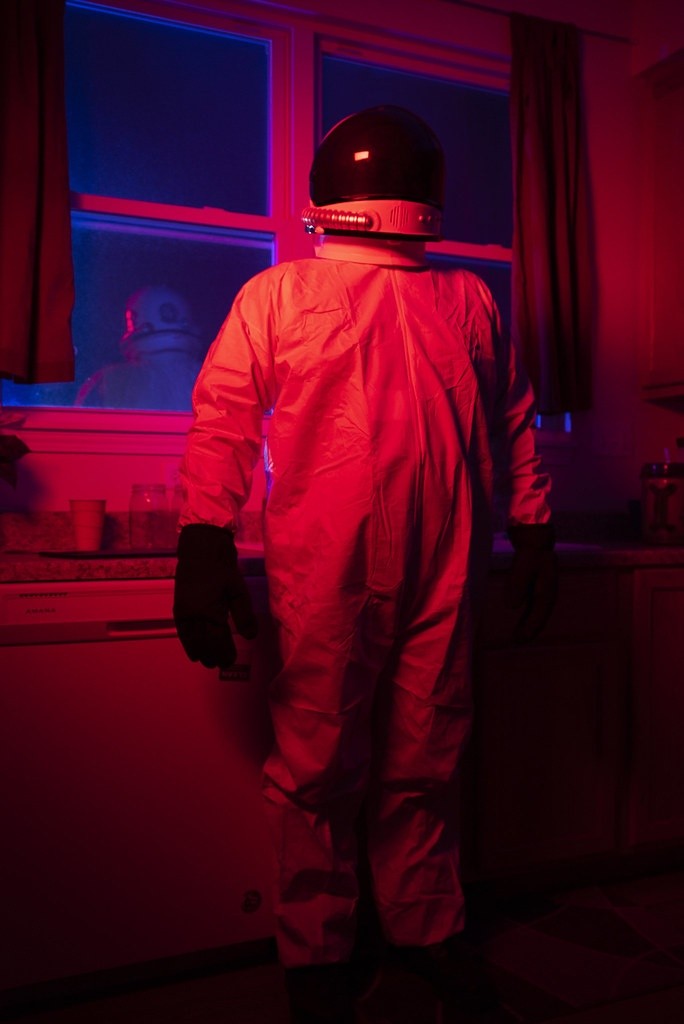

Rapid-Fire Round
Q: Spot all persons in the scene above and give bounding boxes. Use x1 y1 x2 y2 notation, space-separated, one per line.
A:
170 102 559 1024
74 282 208 413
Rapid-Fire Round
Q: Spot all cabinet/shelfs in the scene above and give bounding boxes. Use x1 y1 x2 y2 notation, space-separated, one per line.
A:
479 566 684 888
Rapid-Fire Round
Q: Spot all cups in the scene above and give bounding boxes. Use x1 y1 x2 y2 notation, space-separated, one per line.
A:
640 462 684 545
69 499 106 550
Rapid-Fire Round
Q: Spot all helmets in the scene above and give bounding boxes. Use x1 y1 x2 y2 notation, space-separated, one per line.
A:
300 105 448 241
115 281 207 363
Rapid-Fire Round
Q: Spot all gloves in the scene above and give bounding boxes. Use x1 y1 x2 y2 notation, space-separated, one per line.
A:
172 522 260 670
508 523 559 644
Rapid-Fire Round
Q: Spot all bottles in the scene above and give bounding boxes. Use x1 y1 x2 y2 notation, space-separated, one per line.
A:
128 484 170 551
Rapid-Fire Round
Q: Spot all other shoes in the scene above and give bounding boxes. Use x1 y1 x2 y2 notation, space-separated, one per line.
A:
401 940 507 1016
287 967 361 1024
